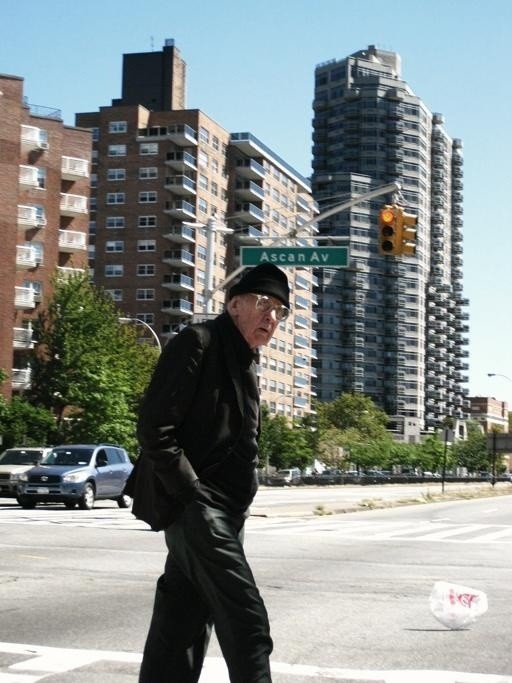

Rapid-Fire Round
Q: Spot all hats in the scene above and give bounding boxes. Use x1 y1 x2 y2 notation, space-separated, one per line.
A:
230 260 290 310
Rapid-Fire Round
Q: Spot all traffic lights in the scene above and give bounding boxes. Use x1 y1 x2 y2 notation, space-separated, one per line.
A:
402 211 418 256
380 209 398 253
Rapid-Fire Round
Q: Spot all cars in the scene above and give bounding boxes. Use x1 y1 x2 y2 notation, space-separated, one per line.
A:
266 466 443 487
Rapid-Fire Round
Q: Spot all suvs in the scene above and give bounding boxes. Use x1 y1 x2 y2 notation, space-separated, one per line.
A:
2 445 51 500
16 442 136 512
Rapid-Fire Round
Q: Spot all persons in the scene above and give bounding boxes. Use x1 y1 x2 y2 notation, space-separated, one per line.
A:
124 262 289 683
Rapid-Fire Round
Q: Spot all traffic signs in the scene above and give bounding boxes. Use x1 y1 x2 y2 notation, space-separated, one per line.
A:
240 244 351 266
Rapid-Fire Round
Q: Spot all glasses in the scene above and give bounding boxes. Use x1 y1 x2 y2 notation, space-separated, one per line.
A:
250 292 290 322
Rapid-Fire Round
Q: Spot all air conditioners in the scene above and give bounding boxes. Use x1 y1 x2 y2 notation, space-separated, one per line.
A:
37 141 50 151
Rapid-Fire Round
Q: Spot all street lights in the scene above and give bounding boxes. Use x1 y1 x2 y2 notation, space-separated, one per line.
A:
488 373 511 384
118 313 164 361
